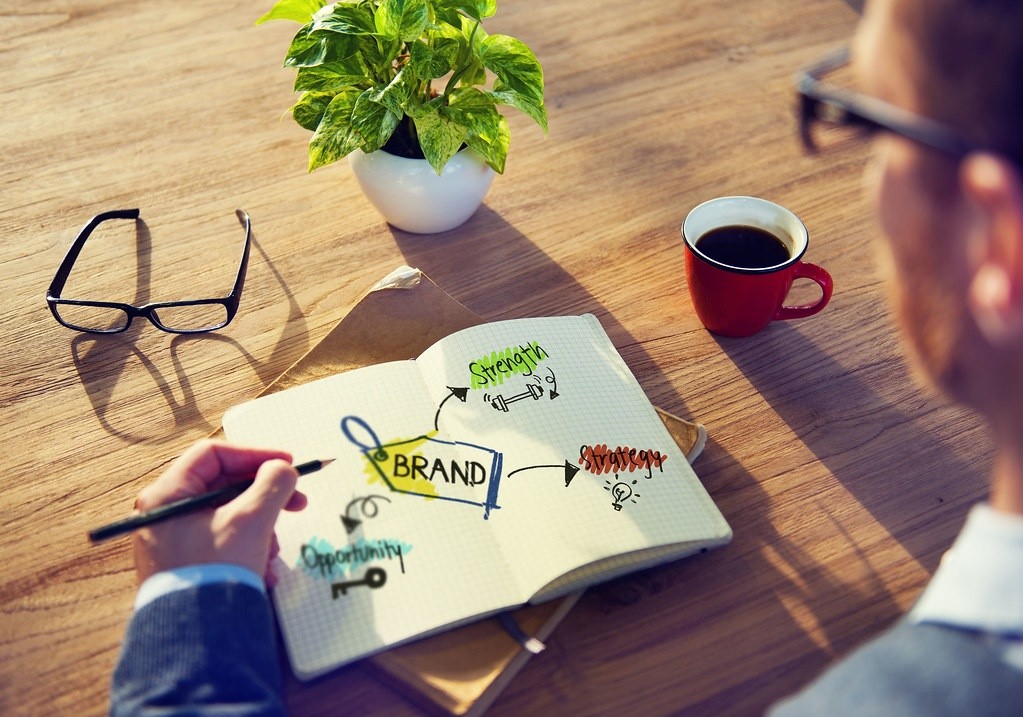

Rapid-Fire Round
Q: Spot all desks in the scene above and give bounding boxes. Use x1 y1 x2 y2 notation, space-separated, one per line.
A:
0 0 998 717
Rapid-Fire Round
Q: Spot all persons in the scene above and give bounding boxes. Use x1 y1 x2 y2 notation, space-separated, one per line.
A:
108 0 1023 717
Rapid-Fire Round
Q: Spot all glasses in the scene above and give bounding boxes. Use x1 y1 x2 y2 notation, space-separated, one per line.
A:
794 47 987 155
44 207 251 336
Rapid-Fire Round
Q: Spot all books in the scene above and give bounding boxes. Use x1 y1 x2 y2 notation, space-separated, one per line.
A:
133 265 734 717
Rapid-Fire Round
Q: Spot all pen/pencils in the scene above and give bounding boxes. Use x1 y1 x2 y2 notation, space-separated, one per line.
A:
87 458 338 543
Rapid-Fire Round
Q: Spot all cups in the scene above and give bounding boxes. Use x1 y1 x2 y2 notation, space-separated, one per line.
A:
681 196 833 338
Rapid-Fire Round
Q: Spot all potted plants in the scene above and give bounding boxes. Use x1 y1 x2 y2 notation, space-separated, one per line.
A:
254 0 548 233
